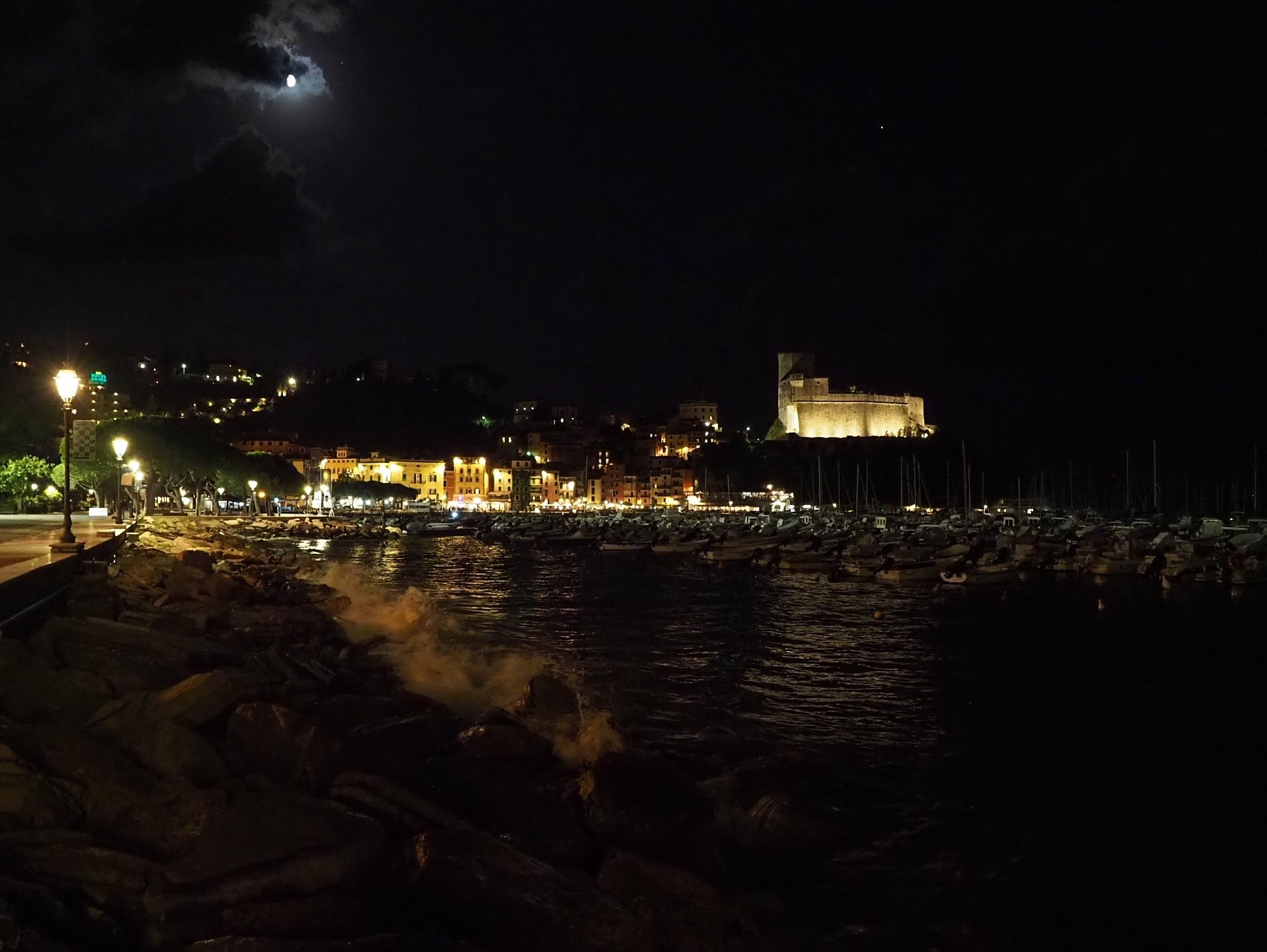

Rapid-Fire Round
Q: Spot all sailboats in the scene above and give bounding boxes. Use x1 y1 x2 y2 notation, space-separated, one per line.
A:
217 441 1266 597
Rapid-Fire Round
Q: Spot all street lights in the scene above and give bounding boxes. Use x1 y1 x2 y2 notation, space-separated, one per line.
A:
45 363 87 553
107 432 131 524
122 453 149 521
